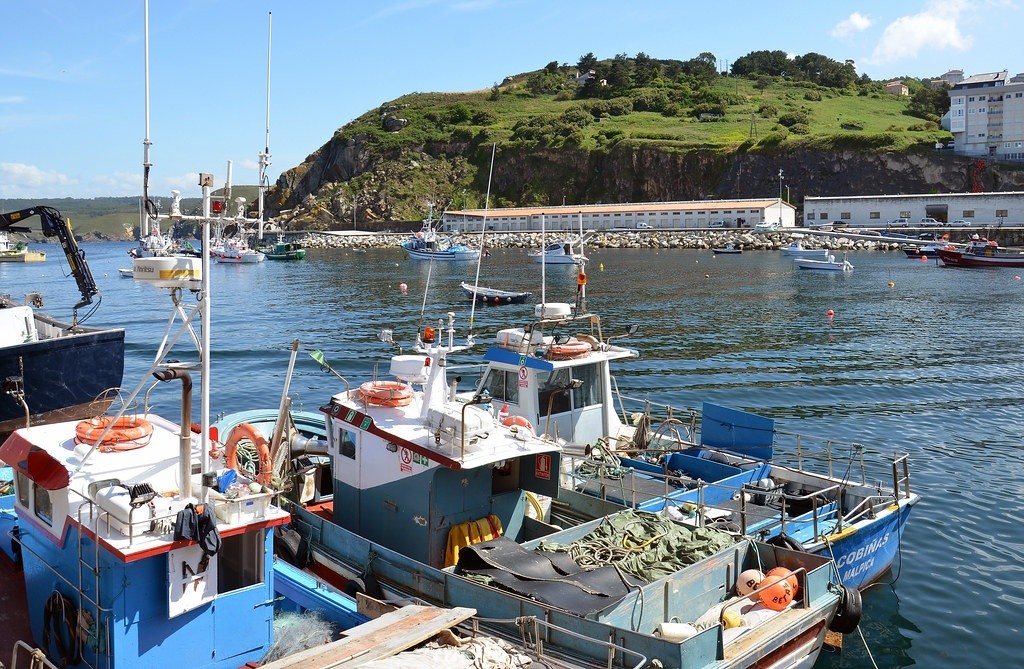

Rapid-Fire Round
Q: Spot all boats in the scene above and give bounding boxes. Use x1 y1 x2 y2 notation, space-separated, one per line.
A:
213 139 843 669
400 194 480 260
527 230 595 265
126 200 305 269
0 1 655 669
459 281 532 302
456 210 922 614
778 241 855 271
712 241 743 254
902 229 1024 268
0 231 46 262
353 247 367 253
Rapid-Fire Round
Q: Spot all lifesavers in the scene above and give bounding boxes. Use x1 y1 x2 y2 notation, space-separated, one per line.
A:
74 416 154 451
546 342 592 359
501 415 534 435
224 422 271 487
359 380 415 407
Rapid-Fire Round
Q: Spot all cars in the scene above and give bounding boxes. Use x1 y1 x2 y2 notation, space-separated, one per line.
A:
918 233 941 241
946 220 971 227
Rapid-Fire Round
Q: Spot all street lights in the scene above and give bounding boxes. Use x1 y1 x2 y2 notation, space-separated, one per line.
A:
778 169 784 227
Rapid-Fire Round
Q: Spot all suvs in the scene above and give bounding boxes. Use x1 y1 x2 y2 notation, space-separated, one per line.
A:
756 222 780 227
921 218 943 227
708 220 726 228
637 223 652 229
887 218 908 228
